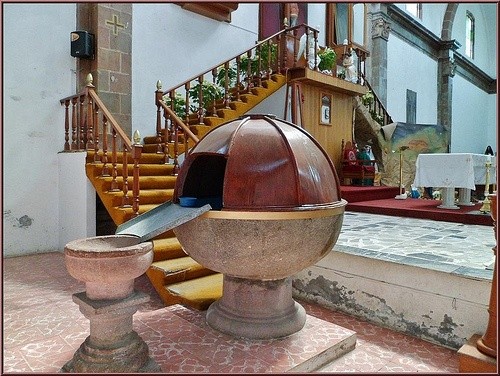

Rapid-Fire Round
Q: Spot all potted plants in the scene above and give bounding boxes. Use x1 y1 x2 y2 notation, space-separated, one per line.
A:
164 42 278 133
318 46 336 76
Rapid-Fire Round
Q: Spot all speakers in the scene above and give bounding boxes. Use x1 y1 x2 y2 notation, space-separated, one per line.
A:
71 31 93 59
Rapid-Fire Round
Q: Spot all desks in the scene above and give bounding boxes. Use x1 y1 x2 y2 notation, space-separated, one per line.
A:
413 153 496 209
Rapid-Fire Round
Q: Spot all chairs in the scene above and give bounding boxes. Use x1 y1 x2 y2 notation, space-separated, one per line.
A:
341 138 378 186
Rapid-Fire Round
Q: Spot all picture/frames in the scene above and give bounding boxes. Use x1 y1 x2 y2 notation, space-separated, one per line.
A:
319 91 333 126
